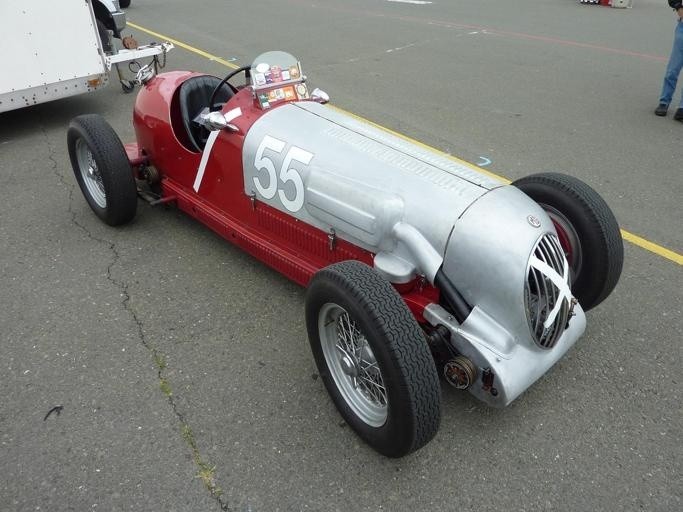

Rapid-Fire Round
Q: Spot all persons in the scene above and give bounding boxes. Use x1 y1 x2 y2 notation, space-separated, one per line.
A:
655 0 683 121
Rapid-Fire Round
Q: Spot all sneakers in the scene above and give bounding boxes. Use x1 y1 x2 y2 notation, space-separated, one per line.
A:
655 104 669 116
674 108 683 121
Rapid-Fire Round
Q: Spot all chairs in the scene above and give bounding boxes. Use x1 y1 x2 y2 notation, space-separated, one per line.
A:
179 74 233 154
